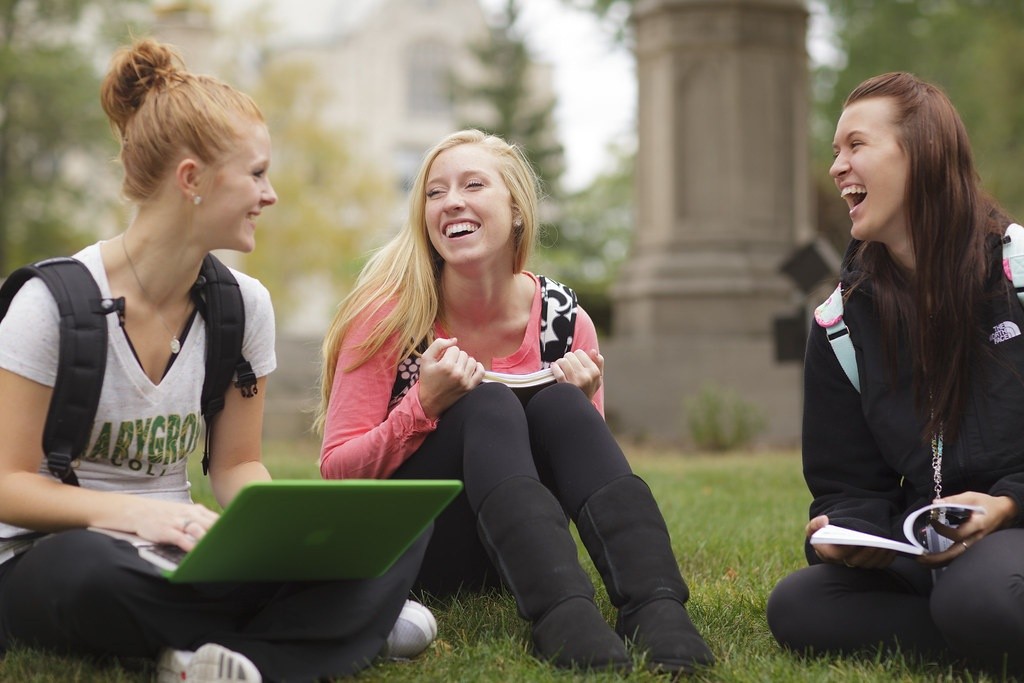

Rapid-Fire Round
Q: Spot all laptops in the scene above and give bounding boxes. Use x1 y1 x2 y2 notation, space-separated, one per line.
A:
84 481 466 586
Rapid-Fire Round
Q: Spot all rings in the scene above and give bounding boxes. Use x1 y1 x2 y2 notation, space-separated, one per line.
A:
179 517 194 531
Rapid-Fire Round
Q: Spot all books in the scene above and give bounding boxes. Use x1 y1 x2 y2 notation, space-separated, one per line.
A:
480 361 557 407
808 498 988 557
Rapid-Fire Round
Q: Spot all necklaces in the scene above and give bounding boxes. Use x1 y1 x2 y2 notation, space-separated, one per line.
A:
958 540 969 553
120 231 194 356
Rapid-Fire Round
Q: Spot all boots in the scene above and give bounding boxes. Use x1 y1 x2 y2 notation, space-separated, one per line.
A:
477 473 633 669
577 475 717 674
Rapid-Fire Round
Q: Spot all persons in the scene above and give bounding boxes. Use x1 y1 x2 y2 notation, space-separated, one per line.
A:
0 36 444 681
309 126 721 676
765 70 1024 676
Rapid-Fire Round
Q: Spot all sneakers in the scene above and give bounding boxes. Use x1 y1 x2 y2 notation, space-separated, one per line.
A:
377 598 440 659
156 642 263 683
